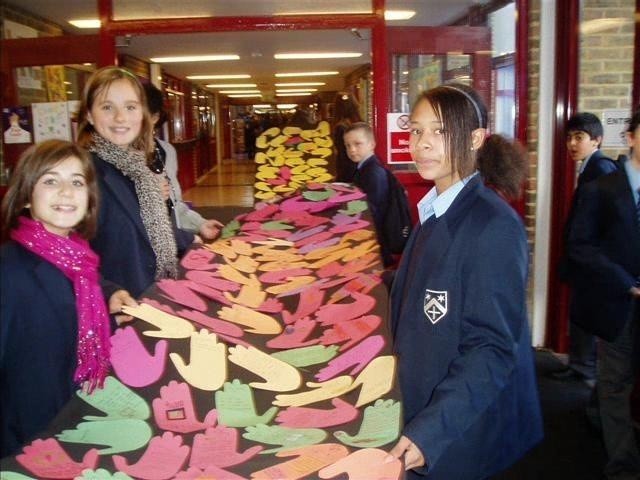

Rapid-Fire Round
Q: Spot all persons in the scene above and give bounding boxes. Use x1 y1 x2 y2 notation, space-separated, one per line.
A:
564 106 640 480
69 66 205 315
139 84 229 246
241 99 361 183
0 135 140 463
342 121 397 269
381 75 546 480
1 112 32 144
547 109 616 384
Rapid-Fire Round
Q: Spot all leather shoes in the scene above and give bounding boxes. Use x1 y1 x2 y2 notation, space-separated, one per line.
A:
550 367 581 379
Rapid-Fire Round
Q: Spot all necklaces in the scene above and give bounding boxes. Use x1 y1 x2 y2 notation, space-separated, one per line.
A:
143 144 180 212
